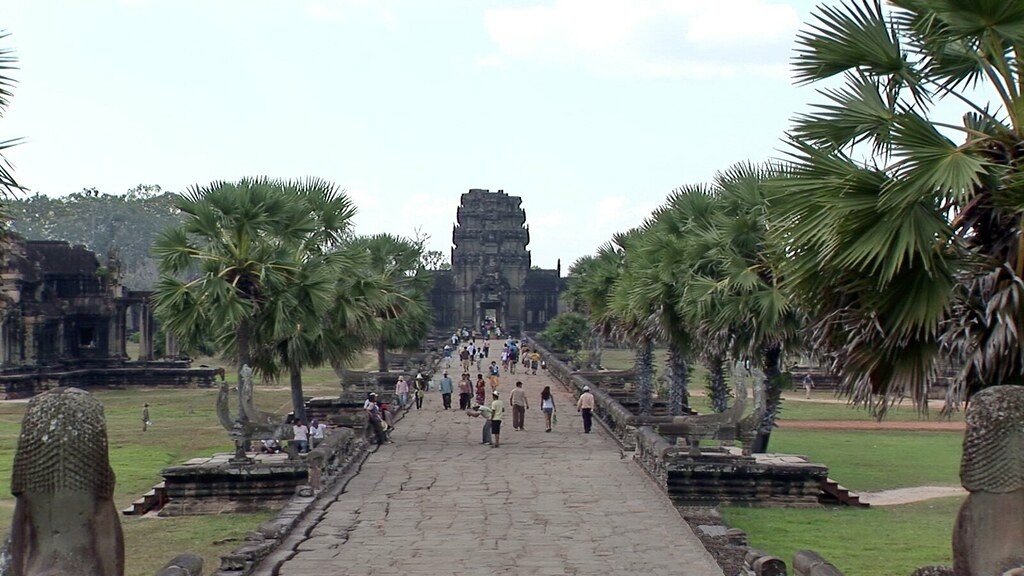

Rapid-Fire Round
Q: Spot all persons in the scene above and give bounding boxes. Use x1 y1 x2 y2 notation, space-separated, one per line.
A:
466 403 492 446
490 390 505 447
308 419 337 451
540 386 556 432
261 440 280 453
509 381 530 431
141 402 150 431
577 385 594 433
364 316 541 445
292 418 309 453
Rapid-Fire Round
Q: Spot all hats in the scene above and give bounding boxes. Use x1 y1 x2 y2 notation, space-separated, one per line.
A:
583 386 591 391
492 391 500 398
370 393 378 397
398 376 404 380
